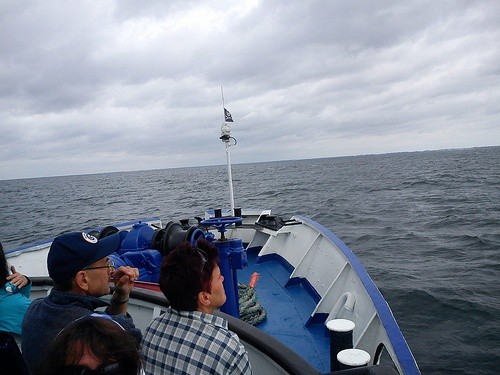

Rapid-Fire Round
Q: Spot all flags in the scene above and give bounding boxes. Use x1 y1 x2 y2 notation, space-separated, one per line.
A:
224 108 234 122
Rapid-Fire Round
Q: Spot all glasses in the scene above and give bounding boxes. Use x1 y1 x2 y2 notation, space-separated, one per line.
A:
194 246 209 273
83 260 115 272
60 361 128 375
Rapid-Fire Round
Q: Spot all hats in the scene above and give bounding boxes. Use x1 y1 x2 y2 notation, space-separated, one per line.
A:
47 230 129 278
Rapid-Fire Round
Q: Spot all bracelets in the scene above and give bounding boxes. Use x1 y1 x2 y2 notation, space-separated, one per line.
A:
111 297 130 305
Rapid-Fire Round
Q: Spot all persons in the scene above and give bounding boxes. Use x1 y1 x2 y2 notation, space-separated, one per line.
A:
142 242 253 375
0 243 33 374
21 230 143 374
38 314 141 374
